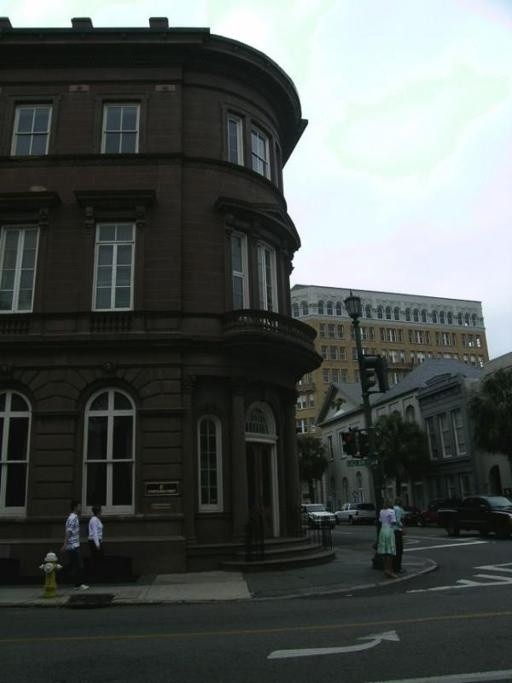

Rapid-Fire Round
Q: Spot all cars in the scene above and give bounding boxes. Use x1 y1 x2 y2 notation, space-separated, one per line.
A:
400 506 419 520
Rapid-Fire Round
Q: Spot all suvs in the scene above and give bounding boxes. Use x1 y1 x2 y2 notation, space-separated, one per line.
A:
415 499 454 527
334 502 378 525
301 504 337 530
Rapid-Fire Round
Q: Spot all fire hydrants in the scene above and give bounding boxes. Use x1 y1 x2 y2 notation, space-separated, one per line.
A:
39 552 63 598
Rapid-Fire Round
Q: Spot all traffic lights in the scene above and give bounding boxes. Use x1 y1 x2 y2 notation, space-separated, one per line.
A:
342 431 357 456
357 434 371 452
360 357 377 391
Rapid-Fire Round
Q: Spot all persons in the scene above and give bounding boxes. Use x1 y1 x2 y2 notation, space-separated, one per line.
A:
378 500 400 580
372 492 385 542
58 502 85 585
391 498 411 565
85 503 108 583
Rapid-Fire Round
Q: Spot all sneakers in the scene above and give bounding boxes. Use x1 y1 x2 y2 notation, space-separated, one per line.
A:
73 584 90 591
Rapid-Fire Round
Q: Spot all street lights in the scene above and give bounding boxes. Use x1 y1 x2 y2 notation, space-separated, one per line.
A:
343 289 385 570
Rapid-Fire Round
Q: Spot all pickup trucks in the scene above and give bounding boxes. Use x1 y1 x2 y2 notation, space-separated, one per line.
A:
437 495 512 538
